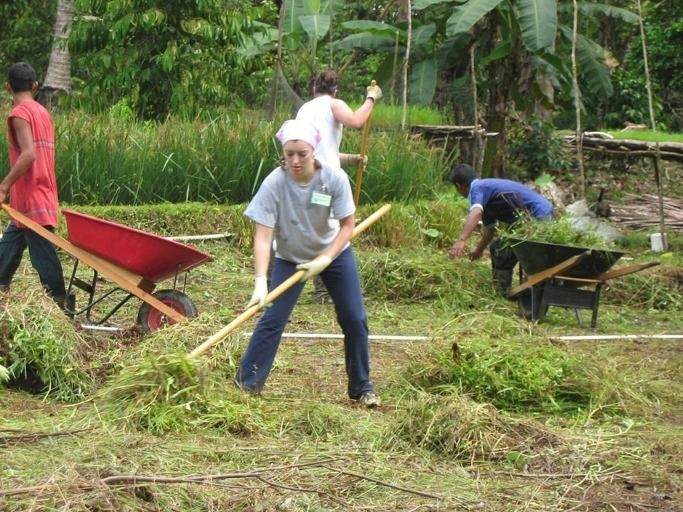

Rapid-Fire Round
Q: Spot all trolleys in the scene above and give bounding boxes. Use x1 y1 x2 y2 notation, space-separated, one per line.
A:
502 233 663 329
1 202 216 339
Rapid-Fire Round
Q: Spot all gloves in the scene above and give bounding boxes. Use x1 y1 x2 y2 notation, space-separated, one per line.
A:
247 272 268 310
348 153 368 167
295 253 332 281
367 84 382 102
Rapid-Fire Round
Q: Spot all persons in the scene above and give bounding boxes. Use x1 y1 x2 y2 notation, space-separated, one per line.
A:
233 119 381 408
448 164 554 296
0 62 69 316
297 72 383 302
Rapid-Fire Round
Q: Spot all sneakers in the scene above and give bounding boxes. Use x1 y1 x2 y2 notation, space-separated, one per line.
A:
362 388 380 407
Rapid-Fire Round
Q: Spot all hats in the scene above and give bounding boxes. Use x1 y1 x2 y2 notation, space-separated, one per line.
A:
277 119 322 150
